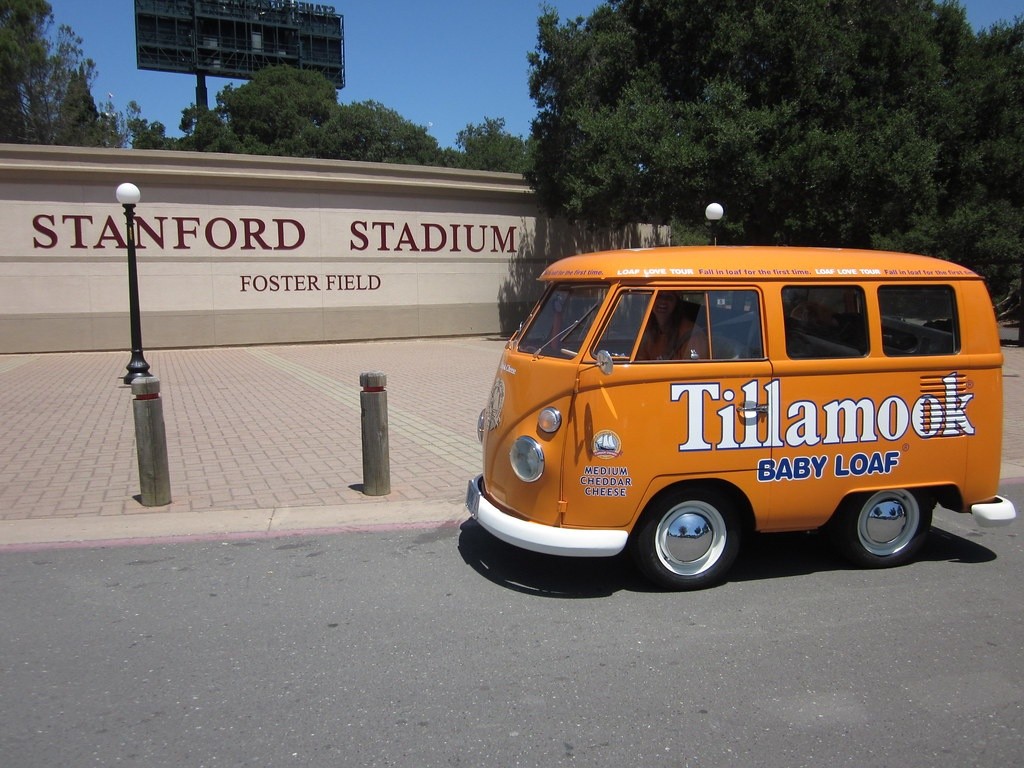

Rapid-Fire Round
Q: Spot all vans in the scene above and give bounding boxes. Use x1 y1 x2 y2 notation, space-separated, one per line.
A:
467 246 1005 593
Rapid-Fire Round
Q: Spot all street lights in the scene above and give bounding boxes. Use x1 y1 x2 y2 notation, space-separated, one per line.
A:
704 202 725 246
115 182 158 386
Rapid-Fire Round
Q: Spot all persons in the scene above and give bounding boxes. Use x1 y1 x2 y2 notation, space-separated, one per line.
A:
631 290 714 360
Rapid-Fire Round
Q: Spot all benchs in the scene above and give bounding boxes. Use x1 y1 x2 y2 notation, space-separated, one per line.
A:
786 329 860 359
848 312 954 356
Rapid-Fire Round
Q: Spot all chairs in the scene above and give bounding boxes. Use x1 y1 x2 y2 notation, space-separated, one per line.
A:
711 333 751 359
